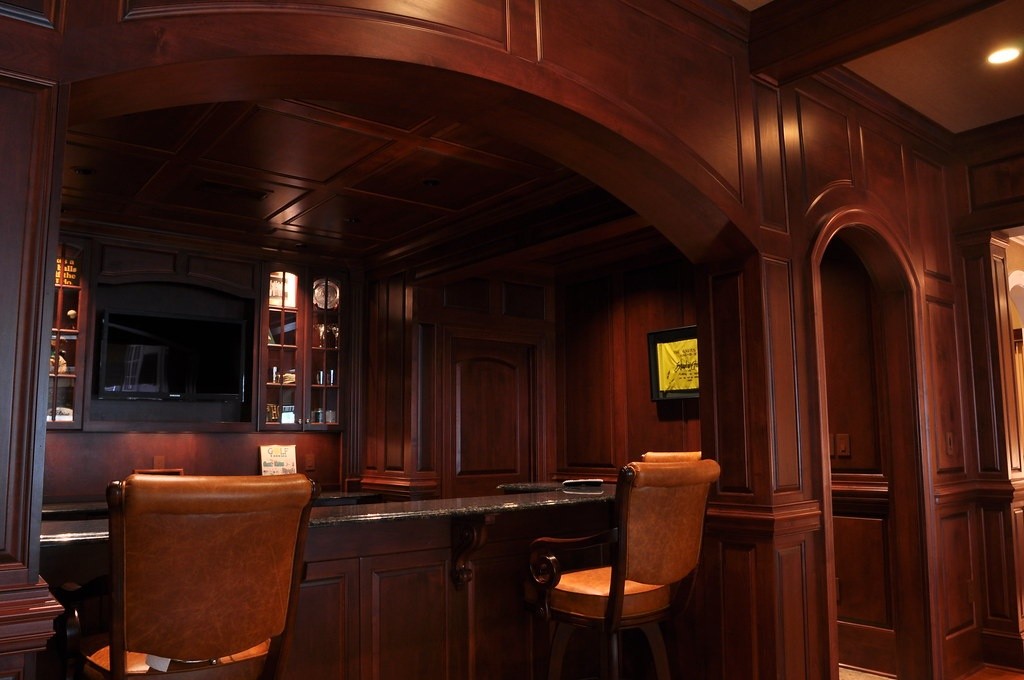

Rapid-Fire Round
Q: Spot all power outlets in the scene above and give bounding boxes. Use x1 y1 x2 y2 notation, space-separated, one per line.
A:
305 454 316 471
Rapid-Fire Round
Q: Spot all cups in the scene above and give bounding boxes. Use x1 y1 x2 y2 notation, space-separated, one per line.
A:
268 366 279 383
326 409 336 423
316 371 324 384
327 369 337 385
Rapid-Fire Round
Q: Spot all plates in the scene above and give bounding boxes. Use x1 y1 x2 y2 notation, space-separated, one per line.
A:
495 482 563 493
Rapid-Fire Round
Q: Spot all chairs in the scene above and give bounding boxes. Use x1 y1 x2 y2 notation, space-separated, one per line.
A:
526 460 722 680
54 472 323 680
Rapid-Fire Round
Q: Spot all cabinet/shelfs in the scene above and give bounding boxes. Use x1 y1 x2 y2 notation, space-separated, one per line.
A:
256 258 351 433
46 233 92 432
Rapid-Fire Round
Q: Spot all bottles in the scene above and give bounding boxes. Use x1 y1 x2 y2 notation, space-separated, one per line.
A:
315 407 324 423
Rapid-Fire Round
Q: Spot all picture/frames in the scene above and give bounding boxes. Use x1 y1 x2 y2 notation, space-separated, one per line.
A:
646 324 701 401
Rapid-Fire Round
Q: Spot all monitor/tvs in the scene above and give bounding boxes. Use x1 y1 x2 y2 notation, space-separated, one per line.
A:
97 309 246 402
